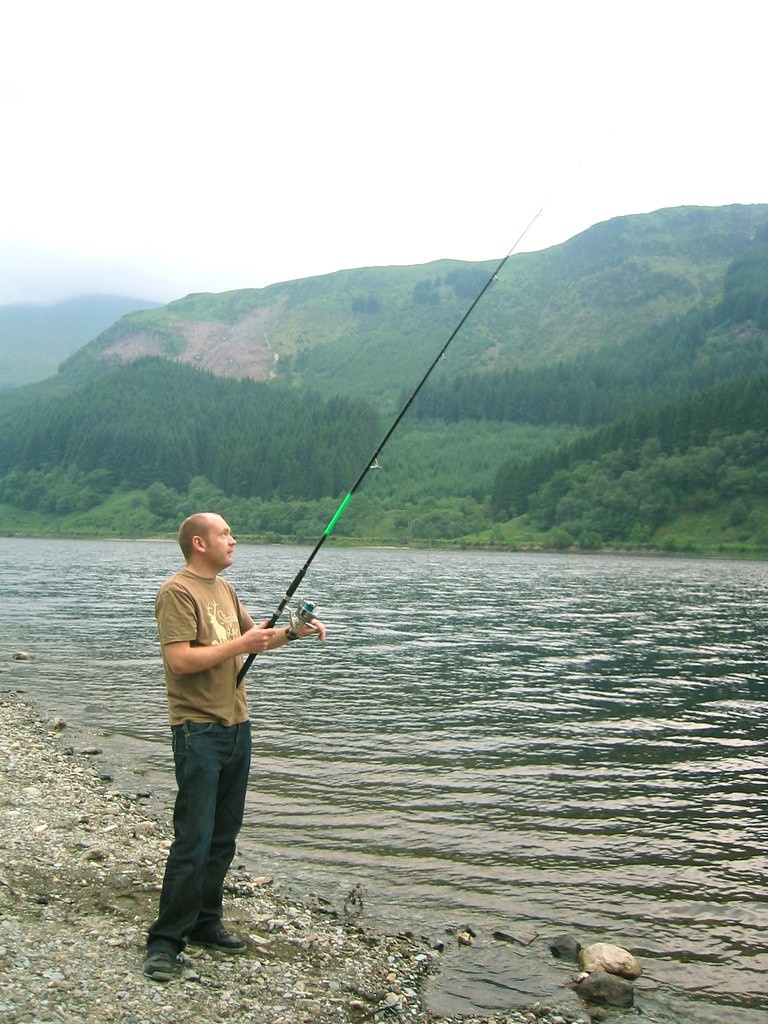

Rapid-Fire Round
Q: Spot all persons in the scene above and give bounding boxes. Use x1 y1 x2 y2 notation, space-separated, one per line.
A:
143 512 327 982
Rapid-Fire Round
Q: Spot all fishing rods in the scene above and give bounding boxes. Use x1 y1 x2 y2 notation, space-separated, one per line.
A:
237 204 548 690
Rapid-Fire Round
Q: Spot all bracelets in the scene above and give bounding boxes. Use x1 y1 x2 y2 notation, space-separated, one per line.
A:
285 624 300 641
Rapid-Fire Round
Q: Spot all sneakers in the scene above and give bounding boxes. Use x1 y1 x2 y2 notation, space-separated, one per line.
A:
143 952 174 981
184 924 246 954
